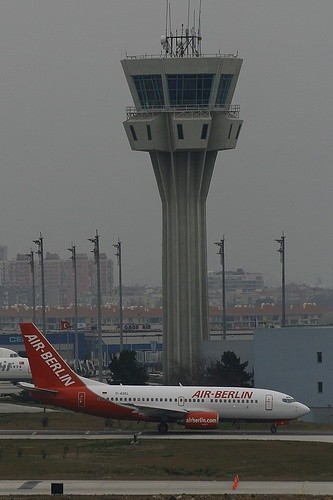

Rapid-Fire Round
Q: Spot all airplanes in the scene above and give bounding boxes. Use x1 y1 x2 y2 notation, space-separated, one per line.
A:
15 322 312 435
0 347 32 386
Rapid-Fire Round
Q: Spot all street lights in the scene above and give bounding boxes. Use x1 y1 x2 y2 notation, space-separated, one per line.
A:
213 234 226 339
31 232 46 337
25 250 36 324
274 230 285 328
66 240 79 374
111 235 123 353
87 229 103 383
